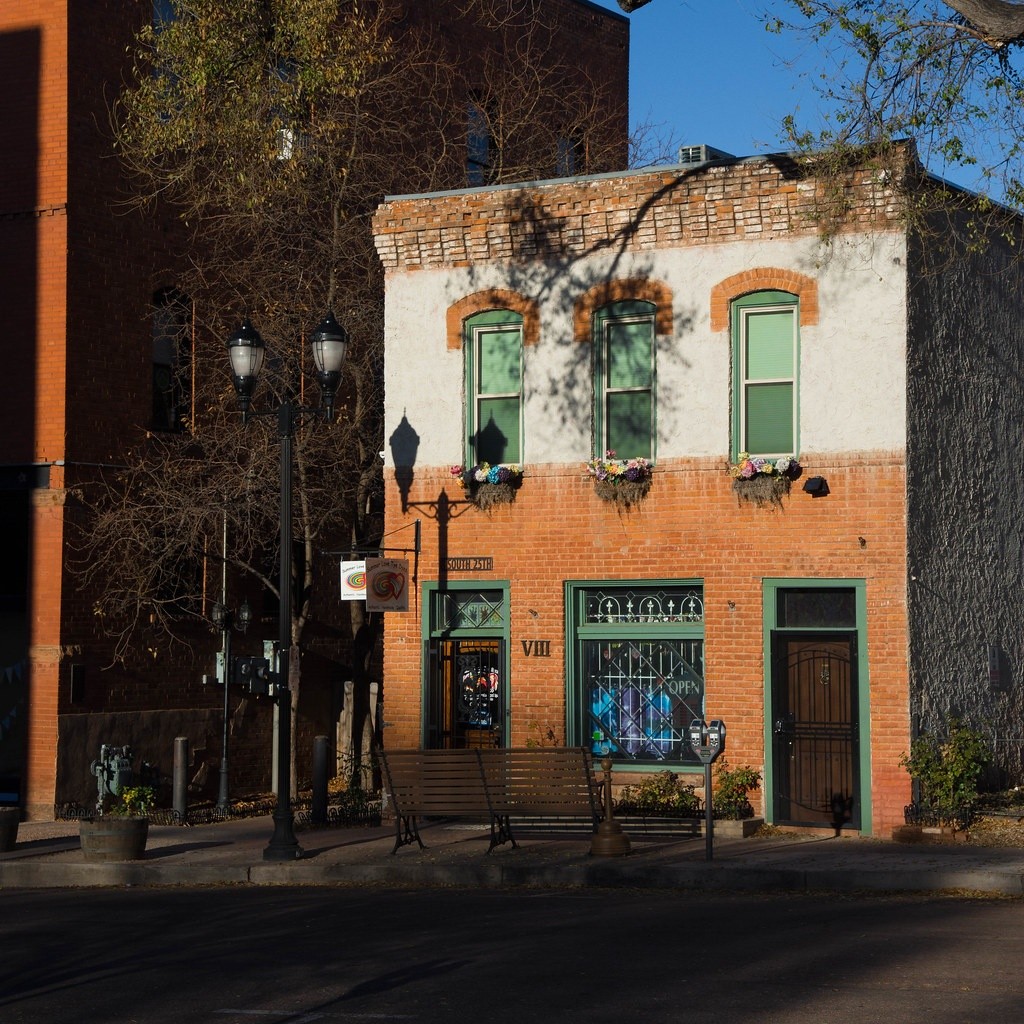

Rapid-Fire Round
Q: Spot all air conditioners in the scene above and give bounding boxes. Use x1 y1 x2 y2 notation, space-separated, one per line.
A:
678 145 737 165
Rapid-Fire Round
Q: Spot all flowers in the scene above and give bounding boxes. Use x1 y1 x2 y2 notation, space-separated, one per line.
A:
106 784 154 816
588 451 655 484
451 464 527 490
731 452 799 481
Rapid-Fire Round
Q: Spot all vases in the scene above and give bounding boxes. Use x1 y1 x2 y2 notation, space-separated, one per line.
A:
78 813 147 862
0 807 21 848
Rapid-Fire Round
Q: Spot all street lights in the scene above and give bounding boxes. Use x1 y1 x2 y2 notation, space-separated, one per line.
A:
224 304 351 866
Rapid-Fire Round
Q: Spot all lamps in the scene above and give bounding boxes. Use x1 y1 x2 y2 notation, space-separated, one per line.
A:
802 476 826 494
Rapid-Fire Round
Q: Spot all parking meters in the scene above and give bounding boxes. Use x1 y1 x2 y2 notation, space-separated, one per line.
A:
694 718 730 872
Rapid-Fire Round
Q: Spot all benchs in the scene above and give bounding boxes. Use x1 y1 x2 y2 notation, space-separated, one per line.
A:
374 744 611 855
